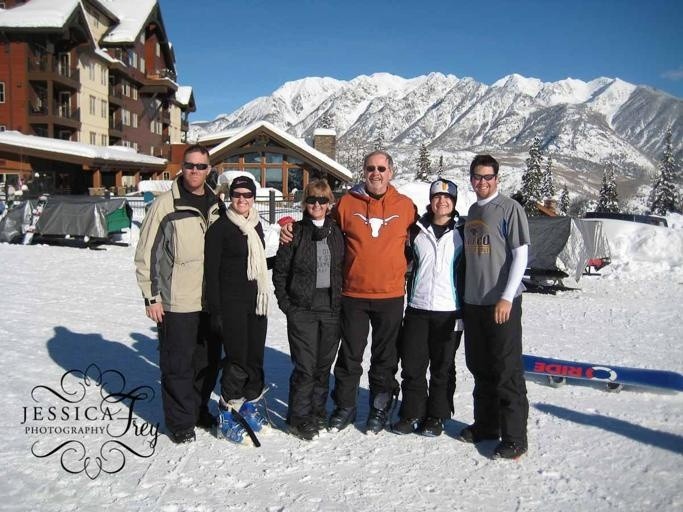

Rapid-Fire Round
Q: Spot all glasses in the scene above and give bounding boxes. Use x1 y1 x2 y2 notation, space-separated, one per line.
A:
305 196 329 204
472 173 496 181
184 163 207 170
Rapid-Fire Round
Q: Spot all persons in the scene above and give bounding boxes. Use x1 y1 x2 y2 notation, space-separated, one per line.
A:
135 145 228 443
458 155 531 461
278 148 419 435
276 177 345 439
205 175 281 445
391 178 466 438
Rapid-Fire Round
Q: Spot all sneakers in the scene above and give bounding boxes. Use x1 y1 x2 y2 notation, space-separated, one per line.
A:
328 405 358 432
197 411 218 428
288 419 320 440
217 395 252 446
420 416 446 437
390 416 426 435
311 414 328 430
239 399 273 434
366 408 389 435
174 426 197 444
494 435 528 459
460 423 500 443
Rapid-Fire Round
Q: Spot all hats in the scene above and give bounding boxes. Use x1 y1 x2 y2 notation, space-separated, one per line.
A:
429 179 457 200
229 176 256 197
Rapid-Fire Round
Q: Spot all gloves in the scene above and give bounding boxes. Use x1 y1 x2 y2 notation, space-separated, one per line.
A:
207 313 226 340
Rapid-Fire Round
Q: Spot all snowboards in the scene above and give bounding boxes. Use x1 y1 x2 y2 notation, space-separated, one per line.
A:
522 355 682 391
